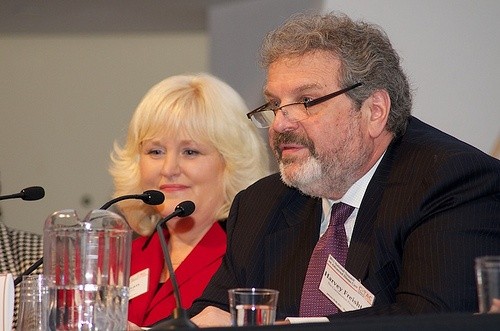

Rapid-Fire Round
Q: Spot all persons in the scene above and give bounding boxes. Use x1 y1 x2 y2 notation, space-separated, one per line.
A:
50 74 268 331
127 10 500 331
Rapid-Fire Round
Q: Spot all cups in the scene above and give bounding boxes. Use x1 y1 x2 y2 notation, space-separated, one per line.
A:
228 288 279 327
475 257 500 314
17 275 57 331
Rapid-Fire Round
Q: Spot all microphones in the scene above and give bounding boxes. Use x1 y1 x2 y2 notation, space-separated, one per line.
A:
147 201 200 331
14 190 165 284
0 186 45 201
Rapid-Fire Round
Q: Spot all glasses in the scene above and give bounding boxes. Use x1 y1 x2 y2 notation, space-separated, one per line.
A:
246 82 363 128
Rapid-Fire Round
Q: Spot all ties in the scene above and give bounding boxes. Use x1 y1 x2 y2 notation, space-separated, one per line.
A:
299 203 355 317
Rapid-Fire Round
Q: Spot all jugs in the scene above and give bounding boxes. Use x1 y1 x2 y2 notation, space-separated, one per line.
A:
41 209 132 330
